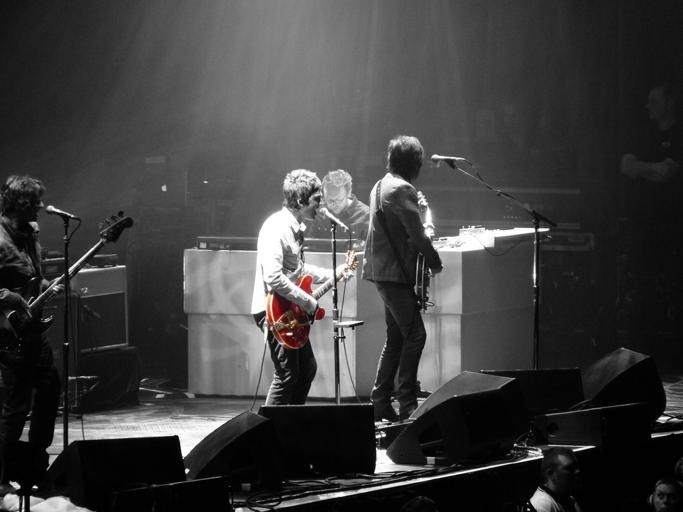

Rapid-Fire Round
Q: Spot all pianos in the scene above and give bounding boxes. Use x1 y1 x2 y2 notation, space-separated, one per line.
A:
195 236 362 252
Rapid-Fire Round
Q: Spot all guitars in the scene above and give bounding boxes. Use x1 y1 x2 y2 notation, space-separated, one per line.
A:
2 210 137 371
267 252 358 348
414 204 435 309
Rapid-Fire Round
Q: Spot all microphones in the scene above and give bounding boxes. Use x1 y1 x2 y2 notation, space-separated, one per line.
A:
429 153 462 162
46 205 79 222
320 208 351 231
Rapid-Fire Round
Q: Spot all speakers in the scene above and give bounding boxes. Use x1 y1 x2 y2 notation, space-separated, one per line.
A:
185 410 266 491
537 403 642 448
46 434 187 506
387 370 514 465
481 367 583 418
261 402 376 477
583 347 667 432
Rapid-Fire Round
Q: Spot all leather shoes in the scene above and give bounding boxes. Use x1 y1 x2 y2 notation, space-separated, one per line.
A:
372 403 400 422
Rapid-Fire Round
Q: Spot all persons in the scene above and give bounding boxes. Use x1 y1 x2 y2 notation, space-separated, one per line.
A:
251 167 355 404
524 446 588 511
359 134 442 424
646 476 683 511
304 167 371 249
0 174 63 487
